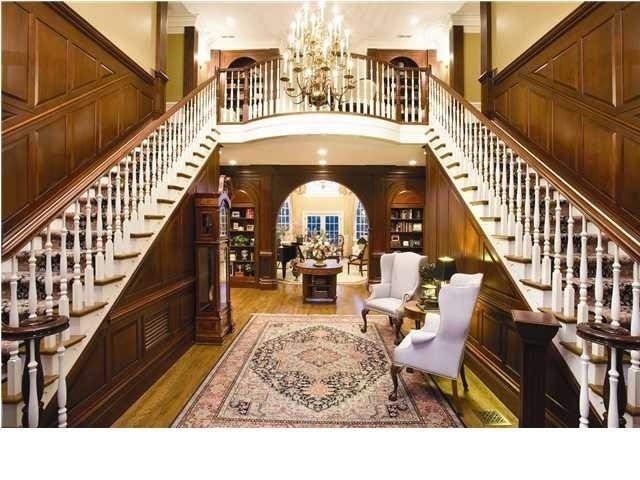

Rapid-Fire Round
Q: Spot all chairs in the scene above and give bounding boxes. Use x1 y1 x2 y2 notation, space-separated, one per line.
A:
349 237 369 276
384 272 483 410
329 234 345 262
361 250 433 346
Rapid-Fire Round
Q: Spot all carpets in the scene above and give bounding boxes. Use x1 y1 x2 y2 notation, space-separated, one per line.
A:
168 313 468 428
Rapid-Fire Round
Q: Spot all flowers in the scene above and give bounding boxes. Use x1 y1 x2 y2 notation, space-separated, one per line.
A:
304 232 336 258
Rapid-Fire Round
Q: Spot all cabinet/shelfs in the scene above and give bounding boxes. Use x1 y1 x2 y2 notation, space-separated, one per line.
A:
367 48 438 124
211 48 279 122
226 205 255 284
385 206 424 255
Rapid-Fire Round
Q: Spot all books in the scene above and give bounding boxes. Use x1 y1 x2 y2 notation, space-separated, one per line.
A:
391 208 423 247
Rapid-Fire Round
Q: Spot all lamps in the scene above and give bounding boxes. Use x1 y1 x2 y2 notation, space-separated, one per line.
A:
276 0 356 110
433 254 458 286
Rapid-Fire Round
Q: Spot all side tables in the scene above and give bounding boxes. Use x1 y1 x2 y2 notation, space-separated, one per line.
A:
404 301 440 373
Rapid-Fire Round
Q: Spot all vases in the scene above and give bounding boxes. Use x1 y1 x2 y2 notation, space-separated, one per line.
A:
312 258 329 268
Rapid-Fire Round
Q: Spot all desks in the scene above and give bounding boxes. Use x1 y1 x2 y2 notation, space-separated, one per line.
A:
297 259 343 304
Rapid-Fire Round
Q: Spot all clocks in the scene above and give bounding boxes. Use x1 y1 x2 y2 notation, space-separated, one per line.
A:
194 192 234 346
